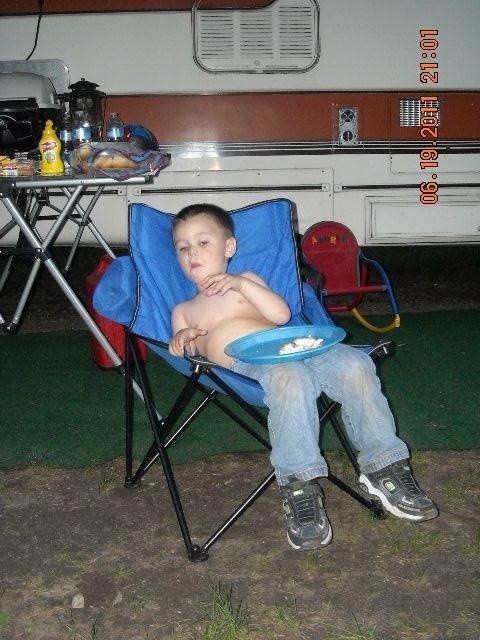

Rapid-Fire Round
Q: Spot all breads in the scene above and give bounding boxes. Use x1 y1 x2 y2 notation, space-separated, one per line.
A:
92 149 138 170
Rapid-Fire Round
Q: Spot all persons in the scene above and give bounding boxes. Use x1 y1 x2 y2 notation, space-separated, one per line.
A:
165 202 442 556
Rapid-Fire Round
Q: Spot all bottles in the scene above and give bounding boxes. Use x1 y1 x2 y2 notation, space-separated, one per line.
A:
38 119 65 177
59 112 124 176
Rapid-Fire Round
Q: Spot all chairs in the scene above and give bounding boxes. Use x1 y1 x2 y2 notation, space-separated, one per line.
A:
92 198 389 563
298 220 401 333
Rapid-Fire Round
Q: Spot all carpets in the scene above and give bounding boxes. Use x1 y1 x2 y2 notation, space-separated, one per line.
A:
0 305 480 466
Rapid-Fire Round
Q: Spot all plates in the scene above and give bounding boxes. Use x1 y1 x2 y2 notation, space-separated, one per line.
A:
223 324 347 365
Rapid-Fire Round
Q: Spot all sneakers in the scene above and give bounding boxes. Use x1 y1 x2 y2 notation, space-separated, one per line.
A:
357 459 438 522
279 478 333 549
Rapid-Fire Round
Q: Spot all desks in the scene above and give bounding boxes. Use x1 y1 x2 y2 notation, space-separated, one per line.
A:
1 153 172 419
